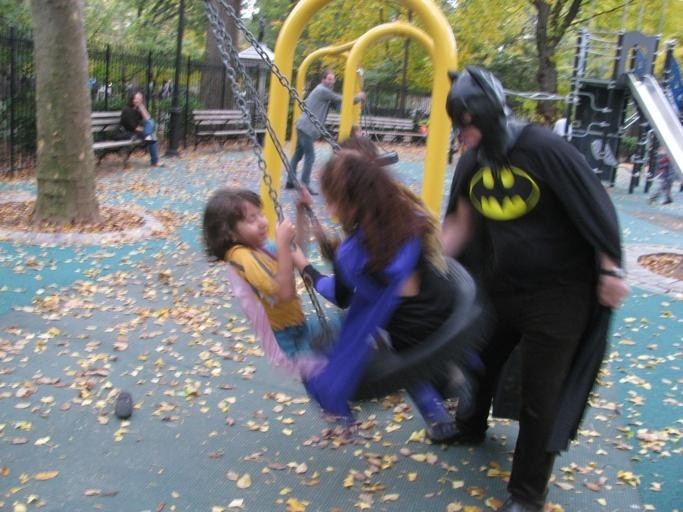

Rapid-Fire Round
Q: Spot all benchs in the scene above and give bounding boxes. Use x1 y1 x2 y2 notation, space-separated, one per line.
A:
192 109 425 152
91 109 153 167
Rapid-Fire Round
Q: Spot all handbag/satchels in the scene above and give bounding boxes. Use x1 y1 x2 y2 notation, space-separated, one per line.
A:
112 126 132 139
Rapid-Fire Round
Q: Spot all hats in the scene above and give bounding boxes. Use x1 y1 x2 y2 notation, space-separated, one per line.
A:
447 66 528 164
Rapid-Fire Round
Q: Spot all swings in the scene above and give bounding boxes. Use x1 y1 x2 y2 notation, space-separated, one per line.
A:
203 4 477 399
346 65 401 166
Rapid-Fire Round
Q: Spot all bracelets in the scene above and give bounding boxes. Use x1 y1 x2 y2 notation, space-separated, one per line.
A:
598 264 627 281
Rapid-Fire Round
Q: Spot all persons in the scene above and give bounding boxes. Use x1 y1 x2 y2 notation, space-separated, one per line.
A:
118 89 164 168
88 76 172 105
647 146 674 206
348 124 364 139
337 133 382 163
198 178 394 382
288 146 489 445
424 63 633 512
285 70 366 196
552 111 574 142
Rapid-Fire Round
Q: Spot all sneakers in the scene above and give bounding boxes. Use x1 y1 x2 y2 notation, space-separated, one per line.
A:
153 162 166 167
144 138 157 144
286 182 318 195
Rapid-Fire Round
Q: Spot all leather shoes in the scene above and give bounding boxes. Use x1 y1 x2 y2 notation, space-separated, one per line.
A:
495 494 542 512
427 422 486 444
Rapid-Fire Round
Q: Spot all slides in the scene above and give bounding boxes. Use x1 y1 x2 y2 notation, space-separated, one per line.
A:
617 71 683 180
